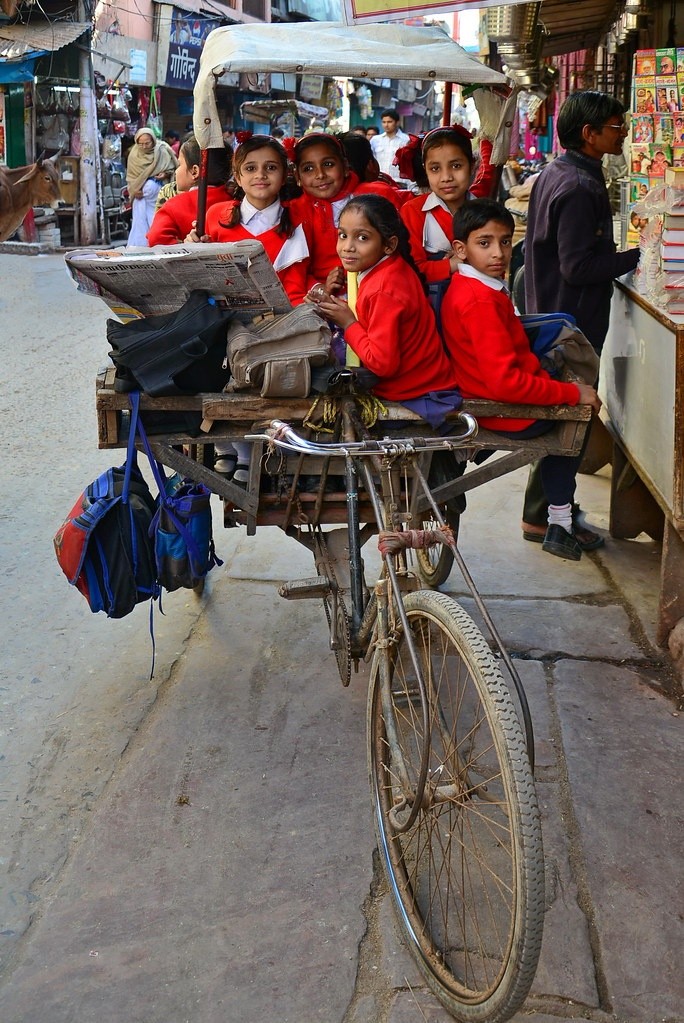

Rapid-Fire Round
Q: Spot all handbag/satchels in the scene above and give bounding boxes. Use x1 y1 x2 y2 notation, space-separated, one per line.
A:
146 85 164 137
34 83 139 165
51 394 165 680
131 391 223 591
311 363 375 392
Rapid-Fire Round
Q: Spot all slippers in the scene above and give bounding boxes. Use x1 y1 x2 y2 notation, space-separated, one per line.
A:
523 522 605 551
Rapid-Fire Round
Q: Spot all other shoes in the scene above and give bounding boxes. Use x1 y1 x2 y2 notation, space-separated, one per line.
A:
542 524 582 561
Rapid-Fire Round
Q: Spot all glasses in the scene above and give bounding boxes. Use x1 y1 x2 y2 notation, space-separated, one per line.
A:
595 123 630 134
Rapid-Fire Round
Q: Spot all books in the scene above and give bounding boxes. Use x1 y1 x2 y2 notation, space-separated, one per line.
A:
627 47 684 245
659 166 684 315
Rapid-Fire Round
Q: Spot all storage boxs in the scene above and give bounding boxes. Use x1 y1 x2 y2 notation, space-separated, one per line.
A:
607 0 643 54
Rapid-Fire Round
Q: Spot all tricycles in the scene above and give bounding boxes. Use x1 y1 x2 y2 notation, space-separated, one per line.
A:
90 20 602 1023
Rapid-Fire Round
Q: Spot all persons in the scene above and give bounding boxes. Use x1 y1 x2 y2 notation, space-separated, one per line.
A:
660 57 674 74
639 216 649 232
125 109 503 488
641 184 648 194
667 90 678 112
169 9 192 46
641 60 654 75
522 91 641 549
439 196 603 561
629 211 640 232
643 90 654 112
638 152 651 174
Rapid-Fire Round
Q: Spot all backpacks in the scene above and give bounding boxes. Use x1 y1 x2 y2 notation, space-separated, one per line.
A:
106 289 231 438
222 302 332 397
518 313 601 387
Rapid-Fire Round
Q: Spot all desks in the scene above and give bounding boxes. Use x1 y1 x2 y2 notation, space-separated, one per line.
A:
596 274 683 542
50 202 132 245
